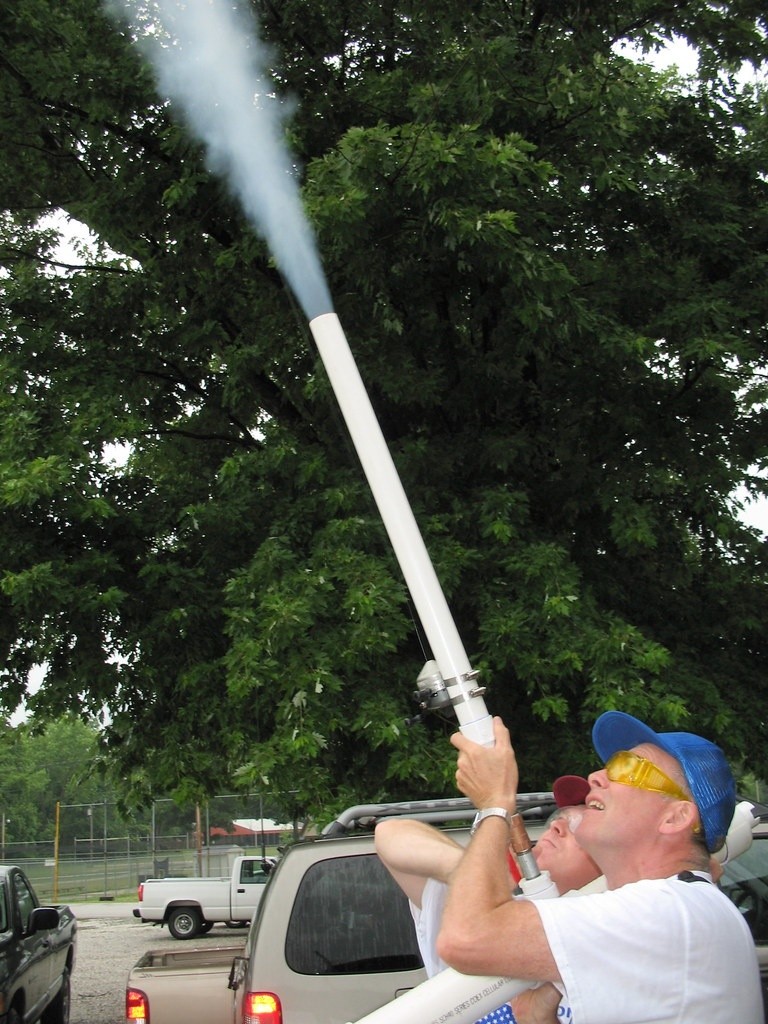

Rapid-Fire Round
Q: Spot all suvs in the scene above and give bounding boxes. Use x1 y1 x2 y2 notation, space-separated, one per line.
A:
229 789 768 1024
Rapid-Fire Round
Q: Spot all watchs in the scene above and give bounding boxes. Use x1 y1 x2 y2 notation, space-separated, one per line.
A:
469 807 512 834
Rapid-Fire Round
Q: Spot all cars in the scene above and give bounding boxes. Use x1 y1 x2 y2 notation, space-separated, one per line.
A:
225 869 268 929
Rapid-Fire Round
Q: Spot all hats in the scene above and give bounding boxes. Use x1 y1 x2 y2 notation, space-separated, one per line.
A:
554 776 591 808
592 711 736 854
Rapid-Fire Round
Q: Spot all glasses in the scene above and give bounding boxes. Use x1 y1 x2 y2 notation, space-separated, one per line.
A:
603 750 701 834
544 805 589 834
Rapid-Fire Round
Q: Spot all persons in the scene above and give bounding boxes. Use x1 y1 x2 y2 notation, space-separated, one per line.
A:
374 712 767 1024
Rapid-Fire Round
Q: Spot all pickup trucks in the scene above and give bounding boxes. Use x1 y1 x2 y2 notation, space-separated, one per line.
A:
0 865 76 1024
133 856 281 940
125 944 245 1024
